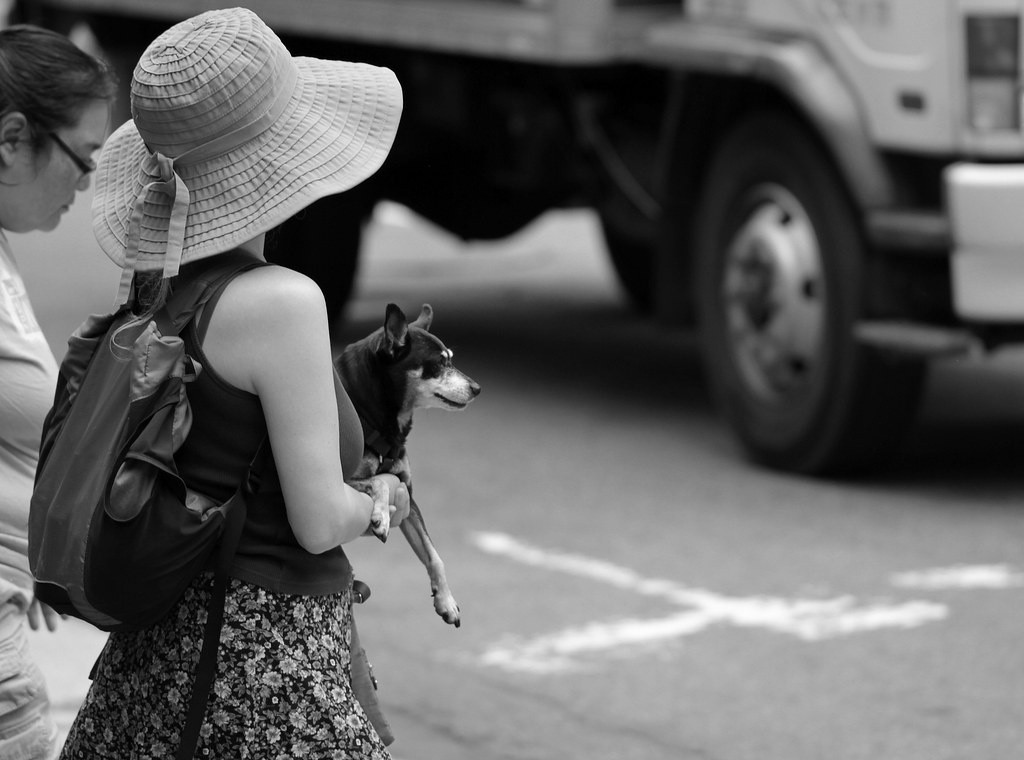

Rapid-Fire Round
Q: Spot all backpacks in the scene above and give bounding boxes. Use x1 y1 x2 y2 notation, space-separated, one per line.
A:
27 256 286 631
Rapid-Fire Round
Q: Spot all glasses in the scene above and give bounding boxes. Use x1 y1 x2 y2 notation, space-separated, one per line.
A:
21 108 97 181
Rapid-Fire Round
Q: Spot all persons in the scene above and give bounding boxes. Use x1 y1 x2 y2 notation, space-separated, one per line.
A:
62 8 412 760
0 23 117 760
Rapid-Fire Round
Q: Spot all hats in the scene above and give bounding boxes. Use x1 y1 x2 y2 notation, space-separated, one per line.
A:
88 7 404 306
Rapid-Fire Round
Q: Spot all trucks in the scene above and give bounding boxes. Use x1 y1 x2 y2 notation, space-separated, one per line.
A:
0 0 1024 475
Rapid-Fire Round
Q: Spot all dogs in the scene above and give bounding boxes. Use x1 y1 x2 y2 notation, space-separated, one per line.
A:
332 302 482 628
